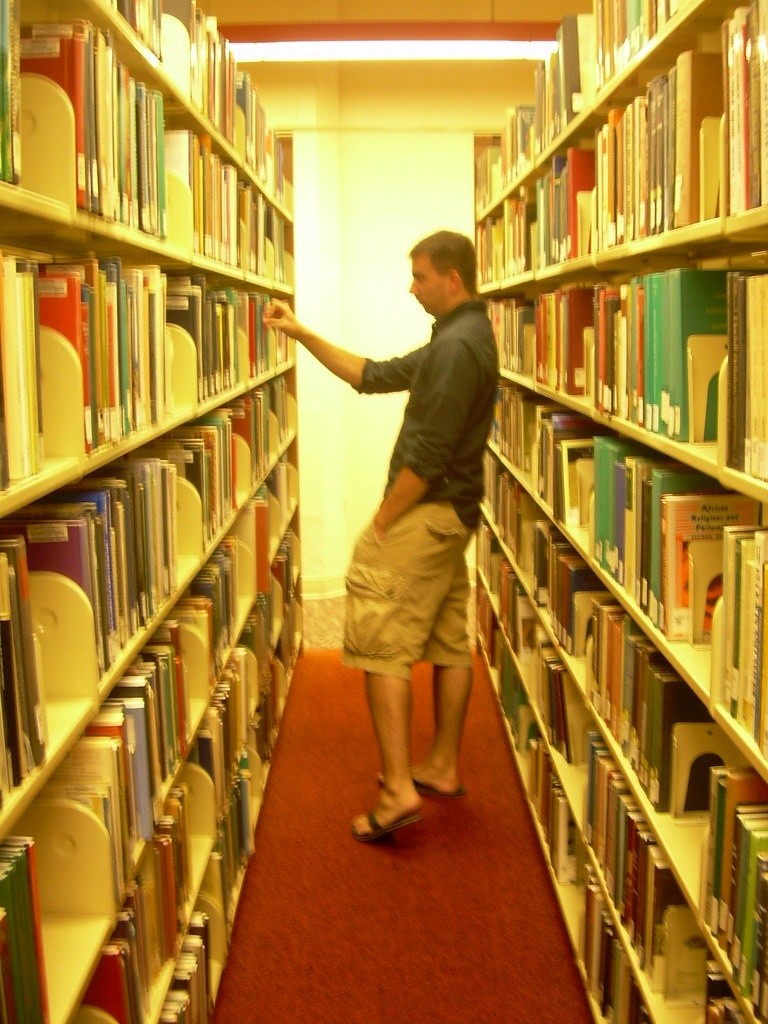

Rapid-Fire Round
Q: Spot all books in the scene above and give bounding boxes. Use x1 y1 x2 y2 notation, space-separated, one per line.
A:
0 0 299 1024
472 0 768 1024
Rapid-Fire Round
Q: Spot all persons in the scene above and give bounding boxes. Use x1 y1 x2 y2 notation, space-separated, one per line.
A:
262 232 500 841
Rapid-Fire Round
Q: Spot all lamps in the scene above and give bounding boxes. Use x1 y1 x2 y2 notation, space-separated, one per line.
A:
214 22 561 64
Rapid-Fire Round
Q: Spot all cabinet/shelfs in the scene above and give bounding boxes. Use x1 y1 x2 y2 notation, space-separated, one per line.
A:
0 1 306 1023
468 1 767 1024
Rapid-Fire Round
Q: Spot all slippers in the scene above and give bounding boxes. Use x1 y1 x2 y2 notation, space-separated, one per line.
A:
379 771 466 798
352 812 423 841
